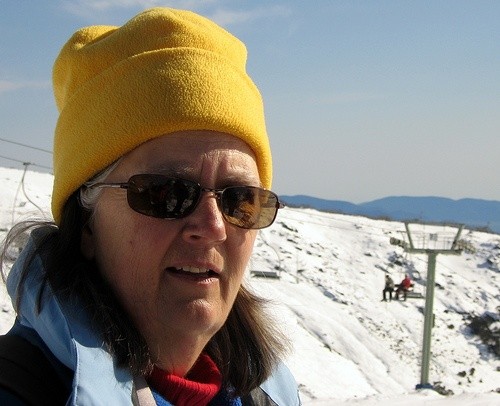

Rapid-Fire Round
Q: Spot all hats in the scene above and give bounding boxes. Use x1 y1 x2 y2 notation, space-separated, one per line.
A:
51 7 274 228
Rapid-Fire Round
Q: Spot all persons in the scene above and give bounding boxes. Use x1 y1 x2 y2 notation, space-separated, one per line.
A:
381 275 396 301
395 274 411 300
228 187 256 218
0 8 301 406
150 178 189 217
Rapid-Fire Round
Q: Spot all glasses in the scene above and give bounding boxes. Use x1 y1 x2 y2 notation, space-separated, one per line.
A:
93 174 286 229
